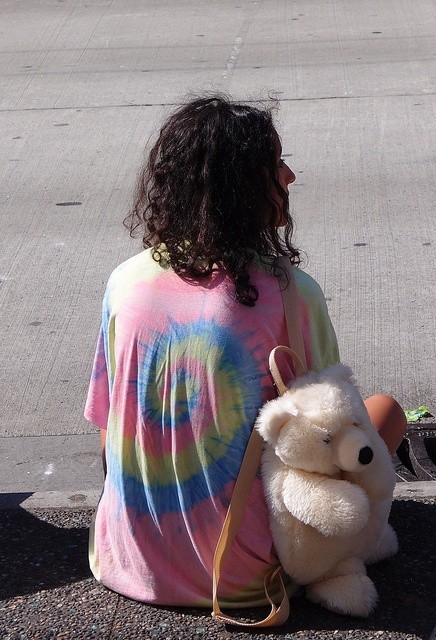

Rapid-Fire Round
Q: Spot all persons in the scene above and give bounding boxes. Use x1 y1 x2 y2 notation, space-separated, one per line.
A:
87 95 407 612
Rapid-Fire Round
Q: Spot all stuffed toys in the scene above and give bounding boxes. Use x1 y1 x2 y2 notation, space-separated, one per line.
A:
255 363 400 617
